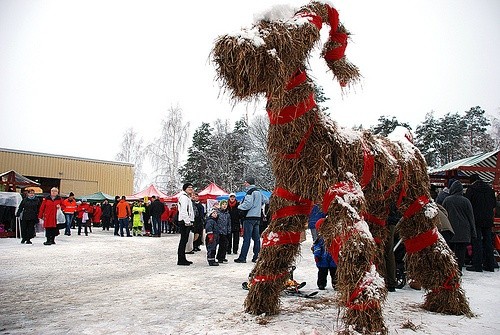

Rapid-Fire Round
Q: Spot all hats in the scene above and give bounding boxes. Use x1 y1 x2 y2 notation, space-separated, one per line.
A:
183 183 193 191
209 207 217 217
469 174 483 184
229 193 236 197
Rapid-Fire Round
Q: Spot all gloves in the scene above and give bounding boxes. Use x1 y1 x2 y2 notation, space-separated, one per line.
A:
208 234 213 243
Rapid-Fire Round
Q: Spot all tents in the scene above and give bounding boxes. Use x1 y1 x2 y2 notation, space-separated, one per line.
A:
195 182 228 204
119 183 178 205
76 190 115 203
216 186 272 199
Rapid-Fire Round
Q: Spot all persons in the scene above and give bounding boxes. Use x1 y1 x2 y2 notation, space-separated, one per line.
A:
15 189 41 244
177 182 194 266
38 187 66 245
61 192 78 236
36 177 270 264
77 197 91 236
309 204 339 292
429 174 500 273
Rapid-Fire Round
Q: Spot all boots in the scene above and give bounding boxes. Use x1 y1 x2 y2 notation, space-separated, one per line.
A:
44 234 55 245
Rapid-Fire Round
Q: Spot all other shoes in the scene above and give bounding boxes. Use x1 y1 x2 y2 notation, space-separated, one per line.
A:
333 285 339 291
121 235 124 237
127 235 132 237
227 251 231 254
26 241 32 244
143 227 178 237
177 260 193 265
137 234 141 236
234 258 246 263
457 254 500 273
233 250 237 254
134 233 136 236
209 257 228 266
396 276 421 290
319 285 326 290
186 251 194 254
254 259 256 262
21 237 27 243
193 248 201 252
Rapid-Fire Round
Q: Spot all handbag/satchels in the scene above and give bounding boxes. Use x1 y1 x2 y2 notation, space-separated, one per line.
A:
56 207 66 224
82 212 89 223
